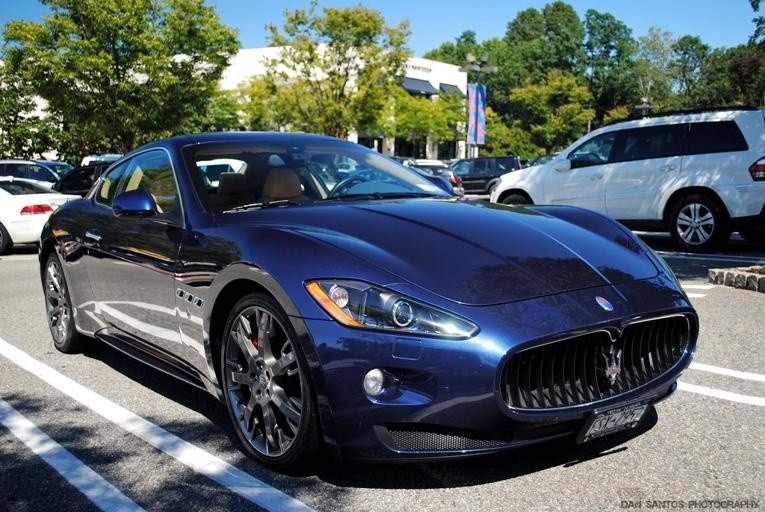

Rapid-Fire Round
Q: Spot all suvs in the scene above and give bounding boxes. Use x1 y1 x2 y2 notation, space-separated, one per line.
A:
489 106 765 250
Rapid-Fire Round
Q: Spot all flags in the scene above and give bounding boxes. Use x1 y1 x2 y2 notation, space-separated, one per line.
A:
465 82 488 146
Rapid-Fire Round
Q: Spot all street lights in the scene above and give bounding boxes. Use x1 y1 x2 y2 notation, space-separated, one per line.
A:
458 52 499 146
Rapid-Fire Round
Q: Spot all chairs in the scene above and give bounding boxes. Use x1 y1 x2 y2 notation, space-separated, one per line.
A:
175 167 310 209
